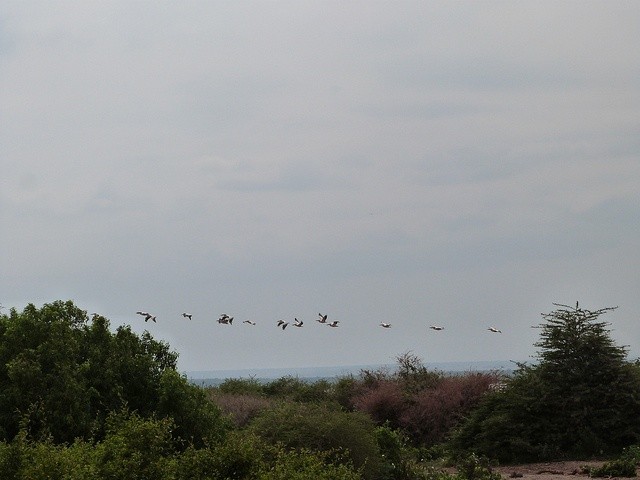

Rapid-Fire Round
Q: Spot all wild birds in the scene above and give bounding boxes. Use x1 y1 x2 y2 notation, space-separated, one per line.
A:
217 313 233 325
316 313 327 323
488 328 501 333
135 311 156 323
327 320 340 327
293 318 303 327
430 326 444 330
380 323 391 328
243 320 256 325
181 312 192 321
276 320 288 330
